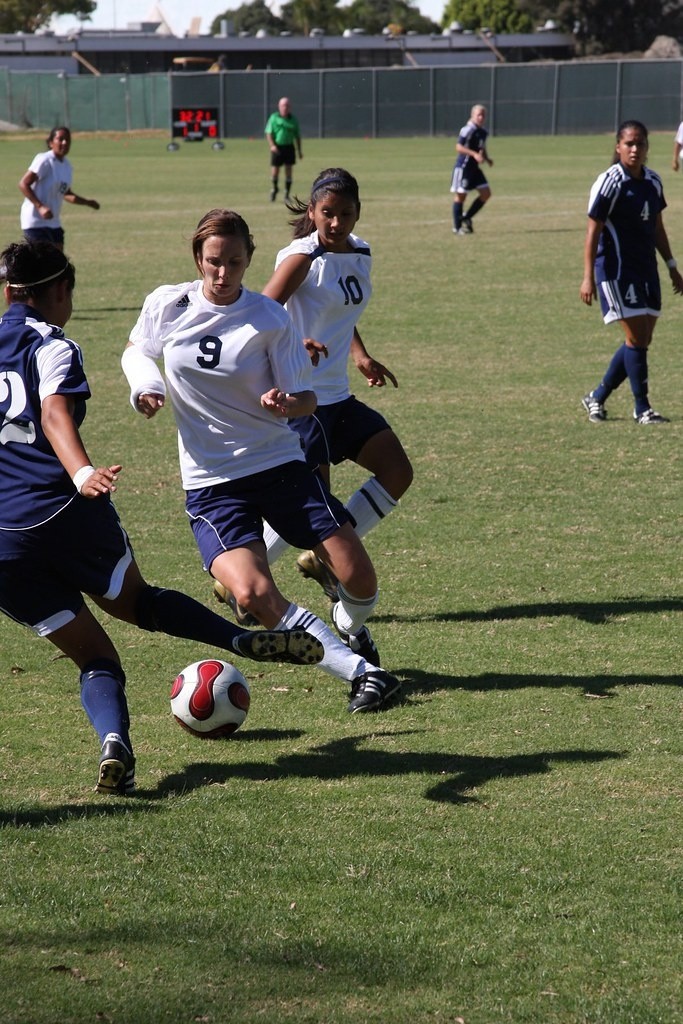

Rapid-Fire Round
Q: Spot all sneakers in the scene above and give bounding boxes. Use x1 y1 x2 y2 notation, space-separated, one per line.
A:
212 578 260 627
634 405 669 425
232 627 324 667
582 387 606 423
94 733 136 796
347 667 401 715
295 548 339 603
330 602 381 669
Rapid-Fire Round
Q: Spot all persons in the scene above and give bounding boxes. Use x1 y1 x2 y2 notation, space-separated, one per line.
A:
449 103 495 236
213 167 414 627
0 233 324 795
580 119 683 425
119 209 403 713
264 95 303 202
17 125 100 254
672 121 683 171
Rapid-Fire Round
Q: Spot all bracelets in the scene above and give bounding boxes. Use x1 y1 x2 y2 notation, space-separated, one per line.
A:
72 465 97 493
667 259 677 269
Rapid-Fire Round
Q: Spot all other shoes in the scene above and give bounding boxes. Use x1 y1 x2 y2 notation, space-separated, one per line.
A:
284 196 292 206
453 226 460 234
462 215 473 233
270 188 279 199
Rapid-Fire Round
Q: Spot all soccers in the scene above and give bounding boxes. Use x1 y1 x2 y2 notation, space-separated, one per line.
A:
169 659 250 739
